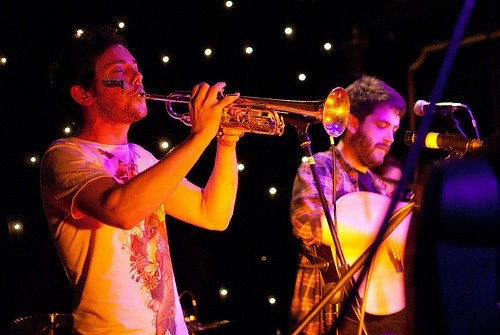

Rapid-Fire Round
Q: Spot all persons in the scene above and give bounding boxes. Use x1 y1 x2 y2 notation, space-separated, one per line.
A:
281 73 438 334
40 34 250 335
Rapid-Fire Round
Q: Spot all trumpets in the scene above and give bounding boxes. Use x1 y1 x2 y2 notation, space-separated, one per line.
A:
137 87 352 144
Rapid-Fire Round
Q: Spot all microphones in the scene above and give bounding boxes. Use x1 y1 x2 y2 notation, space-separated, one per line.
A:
404 99 486 151
